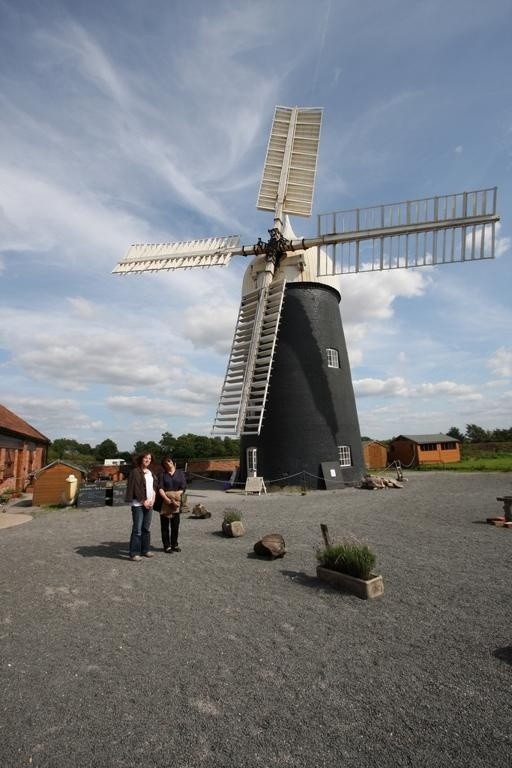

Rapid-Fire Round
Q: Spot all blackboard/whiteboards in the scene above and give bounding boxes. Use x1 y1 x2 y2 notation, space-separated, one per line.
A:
77 482 106 507
245 477 263 492
113 480 128 506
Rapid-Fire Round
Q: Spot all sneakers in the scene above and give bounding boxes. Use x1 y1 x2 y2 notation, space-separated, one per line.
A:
165 548 172 553
129 554 143 562
172 547 181 553
141 550 154 558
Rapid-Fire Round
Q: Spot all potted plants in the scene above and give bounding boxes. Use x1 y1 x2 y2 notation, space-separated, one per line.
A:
221 509 246 538
311 544 385 600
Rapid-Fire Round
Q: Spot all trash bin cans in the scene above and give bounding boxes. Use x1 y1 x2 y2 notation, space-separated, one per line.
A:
64 475 78 504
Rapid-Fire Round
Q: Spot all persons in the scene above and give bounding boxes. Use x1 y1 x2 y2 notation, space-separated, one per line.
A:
124 451 160 561
156 455 187 554
